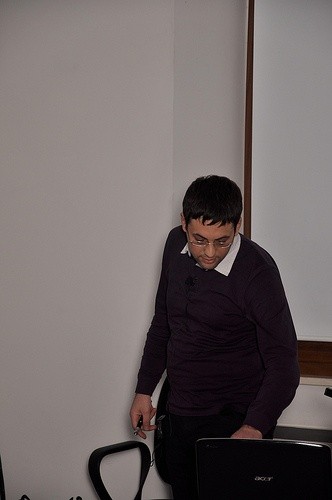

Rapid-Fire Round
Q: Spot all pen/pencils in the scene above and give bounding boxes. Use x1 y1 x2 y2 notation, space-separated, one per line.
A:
134 415 143 436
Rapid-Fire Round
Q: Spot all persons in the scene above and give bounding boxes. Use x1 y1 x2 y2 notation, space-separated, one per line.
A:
130 174 300 484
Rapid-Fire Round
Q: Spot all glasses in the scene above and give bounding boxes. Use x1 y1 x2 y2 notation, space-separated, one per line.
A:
186 228 236 248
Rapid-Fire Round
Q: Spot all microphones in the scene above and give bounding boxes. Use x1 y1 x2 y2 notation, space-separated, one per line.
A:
185 277 193 285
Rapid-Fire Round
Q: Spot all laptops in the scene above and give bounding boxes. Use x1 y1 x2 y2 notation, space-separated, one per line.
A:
196 438 332 500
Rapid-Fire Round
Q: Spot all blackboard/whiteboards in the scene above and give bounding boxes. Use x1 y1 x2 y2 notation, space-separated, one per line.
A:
243 0 332 379
